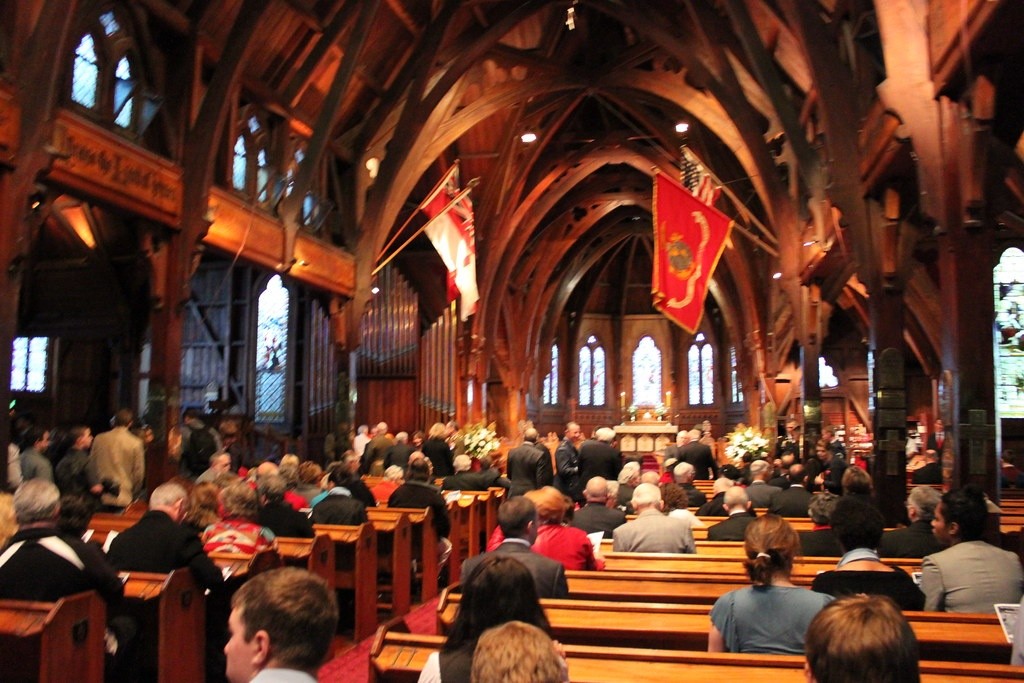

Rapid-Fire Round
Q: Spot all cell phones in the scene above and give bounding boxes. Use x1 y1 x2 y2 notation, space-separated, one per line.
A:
913 572 923 584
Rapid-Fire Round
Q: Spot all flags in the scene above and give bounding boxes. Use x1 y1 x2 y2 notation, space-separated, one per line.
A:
420 158 480 323
679 144 720 209
647 165 736 335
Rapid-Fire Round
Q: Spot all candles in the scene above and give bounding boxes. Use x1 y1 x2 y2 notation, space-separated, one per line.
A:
620 392 625 408
666 391 671 406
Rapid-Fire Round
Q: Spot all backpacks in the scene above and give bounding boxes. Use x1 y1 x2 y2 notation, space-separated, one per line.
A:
181 423 219 477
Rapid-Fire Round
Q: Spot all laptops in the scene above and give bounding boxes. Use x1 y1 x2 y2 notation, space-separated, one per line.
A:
994 603 1020 644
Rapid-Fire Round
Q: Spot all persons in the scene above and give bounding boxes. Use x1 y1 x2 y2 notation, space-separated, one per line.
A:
707 515 835 654
460 419 947 597
921 487 1024 614
11 408 509 582
803 592 920 683
1001 449 1024 488
224 567 337 683
797 486 945 611
0 478 227 683
417 555 569 683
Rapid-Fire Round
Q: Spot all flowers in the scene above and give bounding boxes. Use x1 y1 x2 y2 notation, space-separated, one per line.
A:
458 421 500 459
724 426 770 465
628 405 638 416
656 403 670 416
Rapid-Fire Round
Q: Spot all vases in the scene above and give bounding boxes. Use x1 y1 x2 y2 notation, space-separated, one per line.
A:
658 416 662 421
631 416 635 422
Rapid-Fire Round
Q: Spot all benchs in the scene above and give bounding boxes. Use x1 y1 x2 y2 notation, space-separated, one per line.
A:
369 481 1024 683
0 477 505 683
